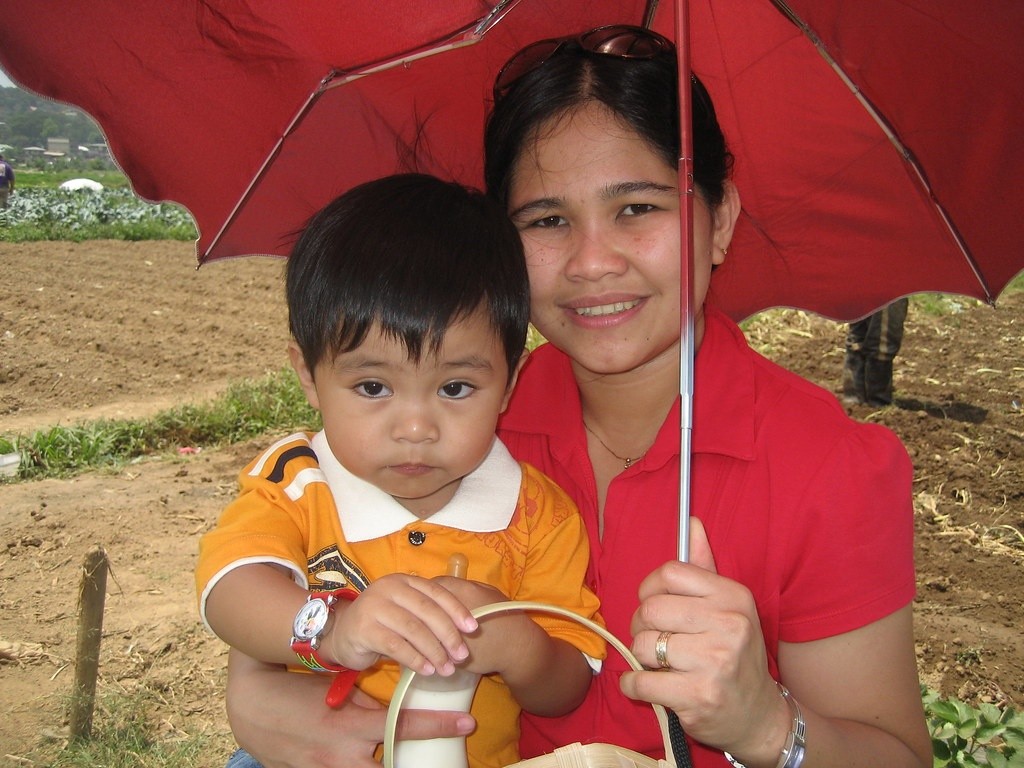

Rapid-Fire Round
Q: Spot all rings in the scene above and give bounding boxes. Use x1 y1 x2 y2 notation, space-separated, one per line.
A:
656 632 673 672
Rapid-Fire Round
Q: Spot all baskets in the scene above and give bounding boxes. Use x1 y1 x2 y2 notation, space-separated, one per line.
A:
381 602 675 768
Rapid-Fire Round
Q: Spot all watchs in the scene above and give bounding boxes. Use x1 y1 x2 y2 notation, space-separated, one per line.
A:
290 589 361 708
724 680 807 768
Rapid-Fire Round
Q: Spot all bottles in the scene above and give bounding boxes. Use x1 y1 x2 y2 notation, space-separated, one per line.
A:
384 553 482 768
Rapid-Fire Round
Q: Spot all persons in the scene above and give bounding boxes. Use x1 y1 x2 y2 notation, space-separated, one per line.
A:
196 23 932 768
0 0 1024 564
0 155 15 208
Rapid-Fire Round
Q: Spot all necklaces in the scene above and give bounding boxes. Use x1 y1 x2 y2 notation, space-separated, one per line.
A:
583 420 647 469
